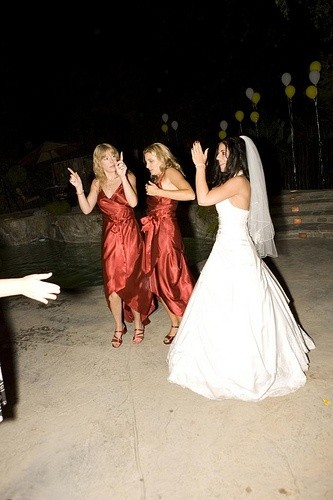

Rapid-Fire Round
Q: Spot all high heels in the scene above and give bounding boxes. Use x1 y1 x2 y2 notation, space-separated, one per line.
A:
112 325 128 347
133 329 145 343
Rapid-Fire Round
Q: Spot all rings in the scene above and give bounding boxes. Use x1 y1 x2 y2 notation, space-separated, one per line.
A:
147 192 149 195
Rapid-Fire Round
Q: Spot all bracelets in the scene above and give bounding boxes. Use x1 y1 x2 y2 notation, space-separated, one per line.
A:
77 190 84 195
195 163 205 168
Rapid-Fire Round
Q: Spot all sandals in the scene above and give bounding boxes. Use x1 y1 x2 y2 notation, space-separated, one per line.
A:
164 326 178 344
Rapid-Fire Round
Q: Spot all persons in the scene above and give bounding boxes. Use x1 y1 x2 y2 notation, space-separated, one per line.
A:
165 136 316 401
0 272 60 422
67 143 195 348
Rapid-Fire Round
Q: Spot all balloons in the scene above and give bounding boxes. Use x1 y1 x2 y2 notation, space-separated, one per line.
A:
162 124 168 132
171 121 178 130
219 88 260 138
306 61 321 99
162 114 168 122
282 73 295 98
